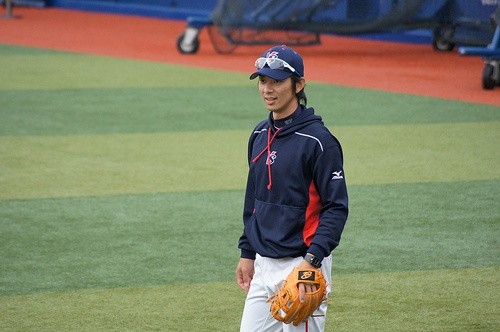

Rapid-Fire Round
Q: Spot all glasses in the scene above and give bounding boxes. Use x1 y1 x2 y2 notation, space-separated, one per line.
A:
254 57 301 77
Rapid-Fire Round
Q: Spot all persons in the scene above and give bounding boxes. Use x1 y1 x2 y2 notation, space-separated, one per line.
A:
234 45 350 332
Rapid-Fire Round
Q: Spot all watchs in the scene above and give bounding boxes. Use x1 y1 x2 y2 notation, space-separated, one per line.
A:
305 253 322 269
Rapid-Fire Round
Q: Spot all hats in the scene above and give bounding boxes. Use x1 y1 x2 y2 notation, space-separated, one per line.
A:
250 45 304 79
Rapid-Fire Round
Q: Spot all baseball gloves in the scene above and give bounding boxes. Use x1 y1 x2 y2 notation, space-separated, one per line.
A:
264 266 330 327
